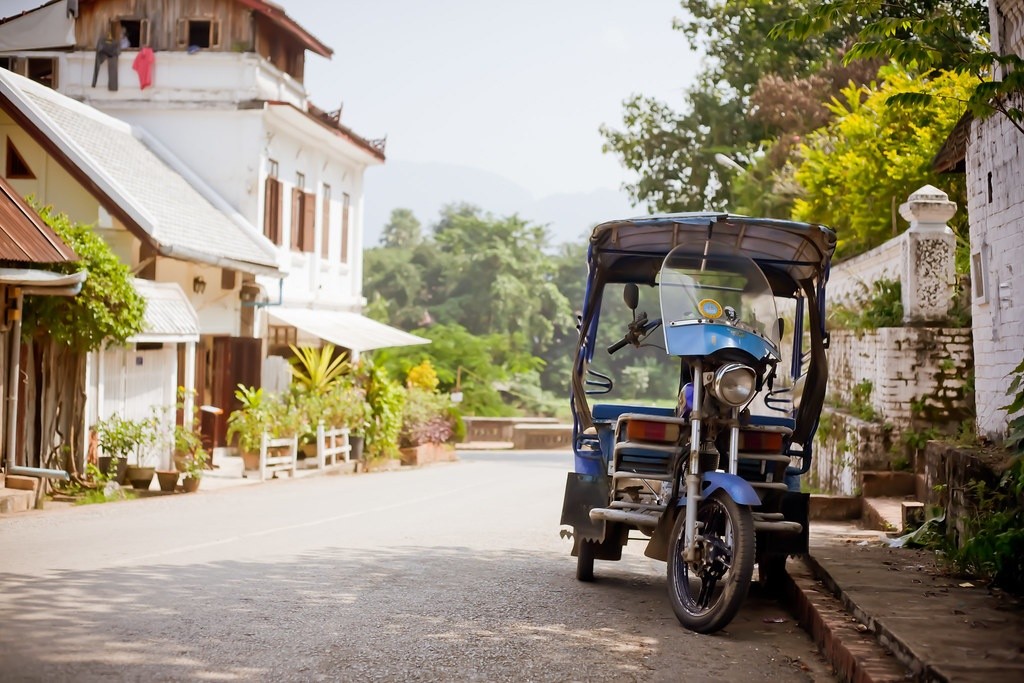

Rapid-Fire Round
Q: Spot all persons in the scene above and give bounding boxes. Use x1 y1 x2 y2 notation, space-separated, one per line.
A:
119 27 130 50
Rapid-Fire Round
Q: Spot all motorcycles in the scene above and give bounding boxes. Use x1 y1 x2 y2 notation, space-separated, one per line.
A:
557 211 840 636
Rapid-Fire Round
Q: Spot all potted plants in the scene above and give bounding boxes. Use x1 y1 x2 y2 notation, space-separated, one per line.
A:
89 386 211 492
226 345 469 473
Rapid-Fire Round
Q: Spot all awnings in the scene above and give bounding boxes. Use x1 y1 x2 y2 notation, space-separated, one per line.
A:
264 302 433 352
